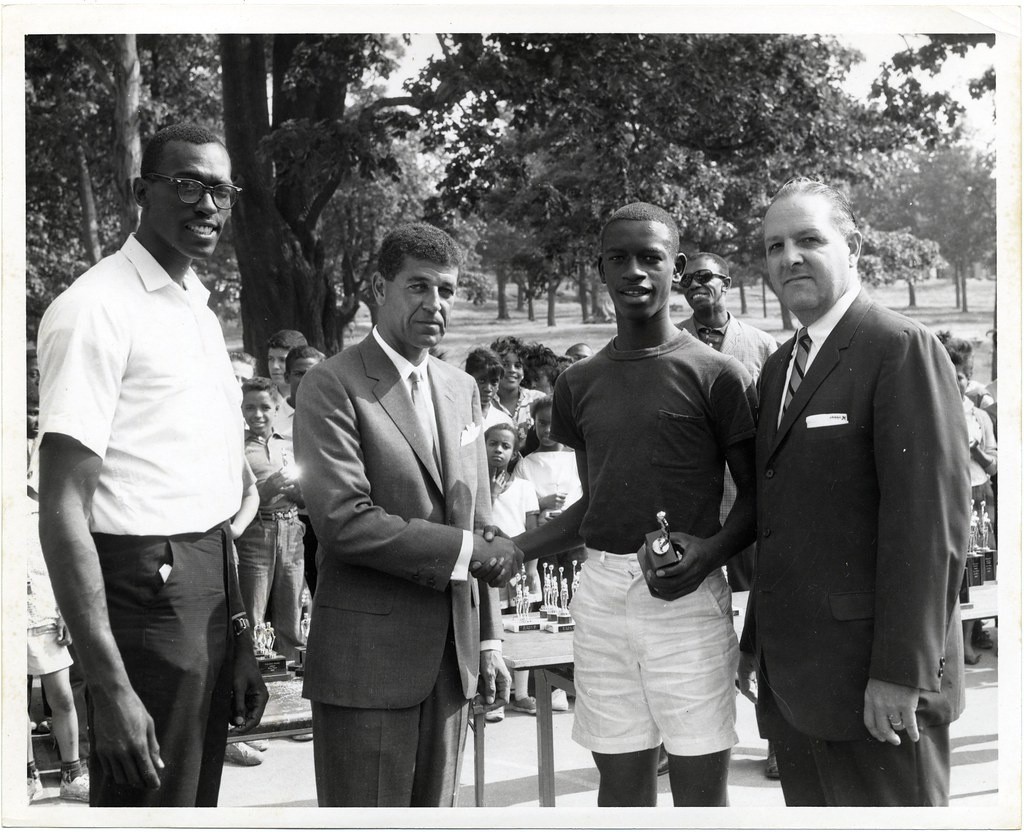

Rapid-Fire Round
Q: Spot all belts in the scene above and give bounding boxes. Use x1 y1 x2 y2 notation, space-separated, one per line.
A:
253 506 298 519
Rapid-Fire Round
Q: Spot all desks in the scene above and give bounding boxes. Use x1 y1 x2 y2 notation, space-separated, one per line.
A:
496 580 997 806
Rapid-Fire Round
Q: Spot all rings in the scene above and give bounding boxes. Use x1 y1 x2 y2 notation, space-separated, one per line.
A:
890 722 901 727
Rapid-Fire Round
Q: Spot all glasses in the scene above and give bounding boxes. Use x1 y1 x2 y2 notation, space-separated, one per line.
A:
144 173 243 210
679 269 727 288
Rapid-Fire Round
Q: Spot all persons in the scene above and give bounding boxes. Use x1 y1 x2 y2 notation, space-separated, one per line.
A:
27 251 996 805
739 180 975 808
295 225 526 807
40 122 248 809
468 200 760 808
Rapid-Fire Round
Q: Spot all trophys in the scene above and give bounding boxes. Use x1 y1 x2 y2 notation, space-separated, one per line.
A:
637 512 680 589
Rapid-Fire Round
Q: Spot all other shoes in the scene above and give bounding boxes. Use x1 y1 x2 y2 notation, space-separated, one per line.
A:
972 630 993 649
224 742 264 765
766 750 779 778
551 688 568 711
59 774 89 802
244 739 269 751
513 696 536 714
485 706 505 722
27 769 43 804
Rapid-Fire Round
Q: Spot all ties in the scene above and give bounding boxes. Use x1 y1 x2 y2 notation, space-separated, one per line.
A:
780 326 811 426
410 370 441 479
